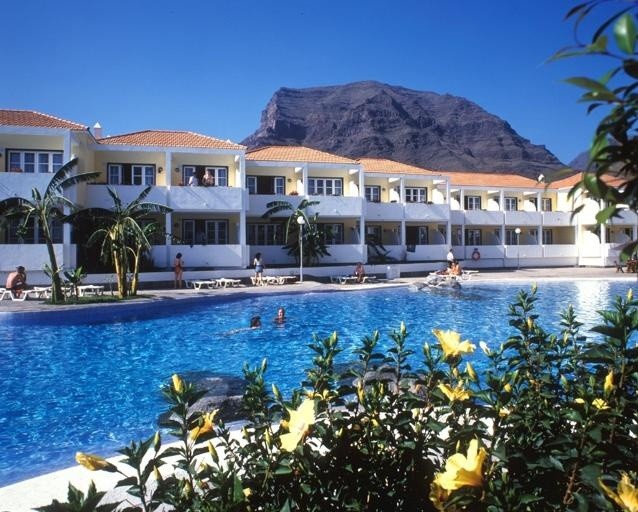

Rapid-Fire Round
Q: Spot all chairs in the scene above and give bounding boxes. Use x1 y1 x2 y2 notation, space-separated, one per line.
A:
614 260 624 272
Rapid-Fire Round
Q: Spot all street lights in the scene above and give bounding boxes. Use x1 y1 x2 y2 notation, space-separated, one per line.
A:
514 227 522 270
296 215 306 282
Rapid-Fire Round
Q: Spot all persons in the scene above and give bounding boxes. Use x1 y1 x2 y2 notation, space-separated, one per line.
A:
253 252 265 286
187 172 198 187
6 266 26 299
447 248 454 268
353 262 364 283
272 307 288 328
202 170 215 187
220 315 261 336
174 252 183 289
436 260 462 276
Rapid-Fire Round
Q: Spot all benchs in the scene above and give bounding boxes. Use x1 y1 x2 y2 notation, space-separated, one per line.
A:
185 277 241 289
330 275 377 284
249 275 297 286
0 285 104 301
429 270 479 281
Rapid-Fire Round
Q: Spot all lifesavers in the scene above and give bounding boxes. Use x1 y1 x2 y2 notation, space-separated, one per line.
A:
472 251 479 261
